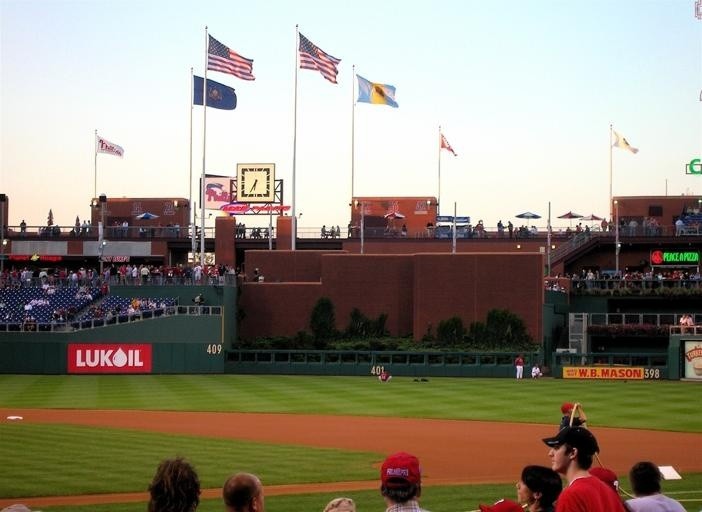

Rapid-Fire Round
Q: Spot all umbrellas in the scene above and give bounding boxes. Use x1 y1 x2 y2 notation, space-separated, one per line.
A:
515 212 541 223
383 212 406 221
557 211 583 222
136 212 159 221
579 215 602 224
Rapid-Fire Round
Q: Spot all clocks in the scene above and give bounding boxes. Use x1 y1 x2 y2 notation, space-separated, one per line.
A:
235 162 276 203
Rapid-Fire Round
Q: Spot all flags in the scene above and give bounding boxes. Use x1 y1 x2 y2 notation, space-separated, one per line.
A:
207 33 255 80
440 133 456 157
612 129 638 154
298 32 341 84
194 75 236 111
356 73 399 108
96 135 125 157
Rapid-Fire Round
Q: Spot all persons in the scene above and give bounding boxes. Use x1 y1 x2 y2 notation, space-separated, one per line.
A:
588 466 619 493
1 264 261 333
532 363 542 379
222 471 265 511
682 315 693 332
148 454 202 511
379 450 433 512
378 369 392 382
514 354 524 382
544 267 701 289
515 464 562 511
559 402 586 432
679 314 685 333
623 461 688 511
541 426 627 511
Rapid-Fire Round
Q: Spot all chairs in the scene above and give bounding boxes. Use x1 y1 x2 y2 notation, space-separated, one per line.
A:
0 284 175 331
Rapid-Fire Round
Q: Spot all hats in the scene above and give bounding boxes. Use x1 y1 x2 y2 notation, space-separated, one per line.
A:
479 498 524 512
561 403 574 413
380 452 421 486
541 426 601 454
589 467 619 491
